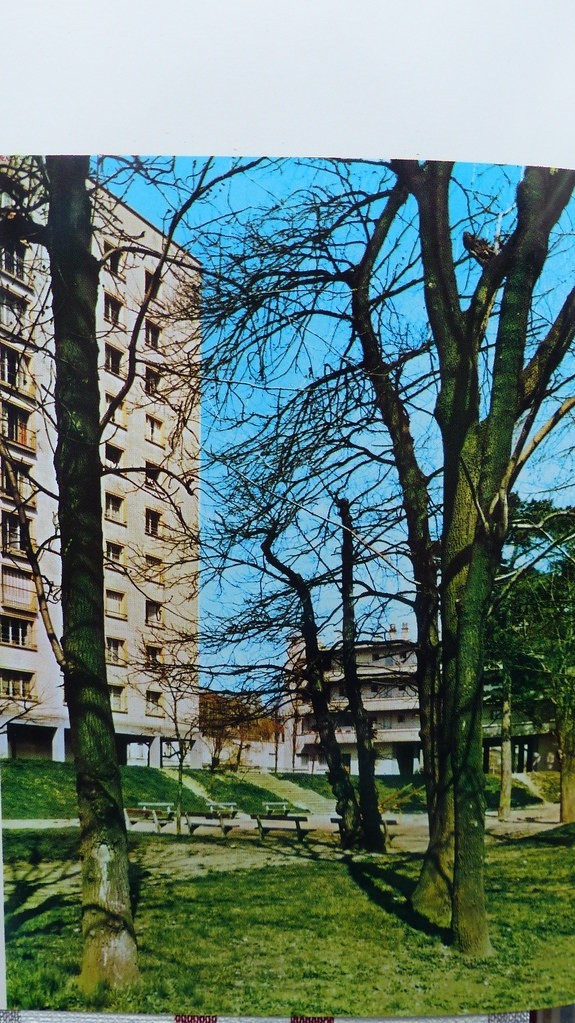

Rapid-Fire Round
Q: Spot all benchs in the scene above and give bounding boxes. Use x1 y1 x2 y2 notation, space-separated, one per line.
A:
250 815 317 844
180 811 240 837
331 818 398 842
139 802 176 820
262 802 295 817
206 802 239 819
123 808 169 837
